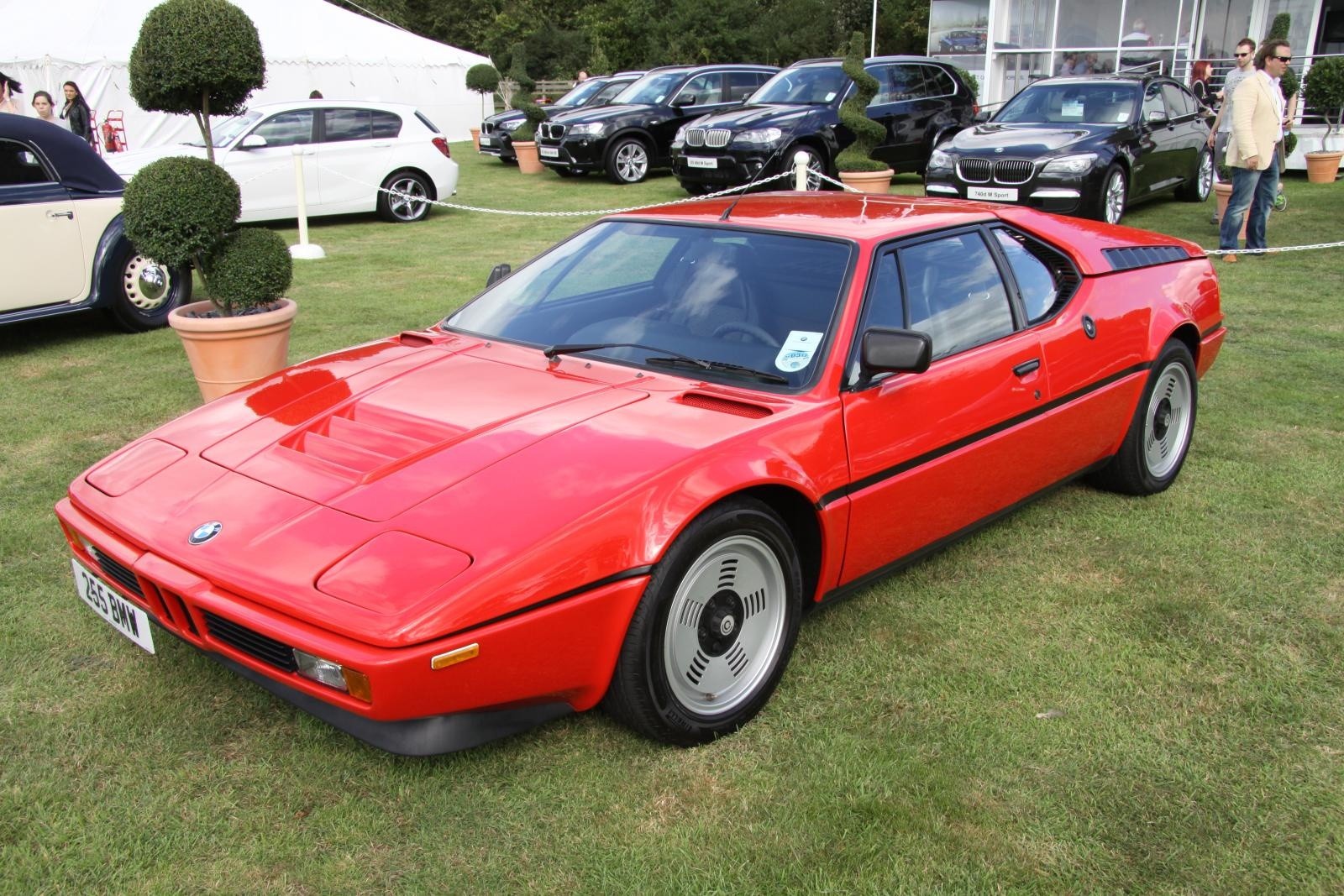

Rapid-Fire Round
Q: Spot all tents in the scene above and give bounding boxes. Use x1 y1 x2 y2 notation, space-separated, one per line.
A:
0 0 494 150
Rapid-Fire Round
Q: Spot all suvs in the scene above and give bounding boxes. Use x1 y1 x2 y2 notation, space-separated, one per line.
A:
475 55 981 194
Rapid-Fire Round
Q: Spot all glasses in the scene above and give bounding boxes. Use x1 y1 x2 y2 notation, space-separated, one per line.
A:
1270 55 1292 63
1234 51 1253 58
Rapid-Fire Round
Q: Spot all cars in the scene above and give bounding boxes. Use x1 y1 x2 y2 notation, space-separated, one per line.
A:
924 72 1219 225
100 100 461 223
54 189 1226 757
0 111 194 334
936 30 987 54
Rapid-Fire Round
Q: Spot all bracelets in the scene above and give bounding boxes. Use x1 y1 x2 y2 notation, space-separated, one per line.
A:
6 98 10 101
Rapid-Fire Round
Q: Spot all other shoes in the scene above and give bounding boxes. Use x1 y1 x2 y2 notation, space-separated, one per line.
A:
1223 252 1236 263
1210 209 1219 224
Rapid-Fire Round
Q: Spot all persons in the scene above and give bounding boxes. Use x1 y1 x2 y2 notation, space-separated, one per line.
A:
32 91 71 131
578 70 590 80
60 81 91 142
1192 62 1223 109
1120 19 1155 74
1062 51 1113 75
309 90 323 99
1260 41 1296 211
1220 41 1291 261
1207 39 1256 224
1167 21 1208 84
0 72 24 115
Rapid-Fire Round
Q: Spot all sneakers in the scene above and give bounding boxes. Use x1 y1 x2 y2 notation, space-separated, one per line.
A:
1274 188 1286 212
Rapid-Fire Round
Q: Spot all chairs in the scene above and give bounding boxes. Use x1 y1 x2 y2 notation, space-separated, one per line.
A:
702 81 720 104
1044 94 1134 124
656 239 942 350
812 80 839 103
269 116 306 145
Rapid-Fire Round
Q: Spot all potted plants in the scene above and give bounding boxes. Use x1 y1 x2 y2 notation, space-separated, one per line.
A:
126 1 297 407
835 30 894 194
508 40 548 175
1214 14 1344 239
466 63 502 150
